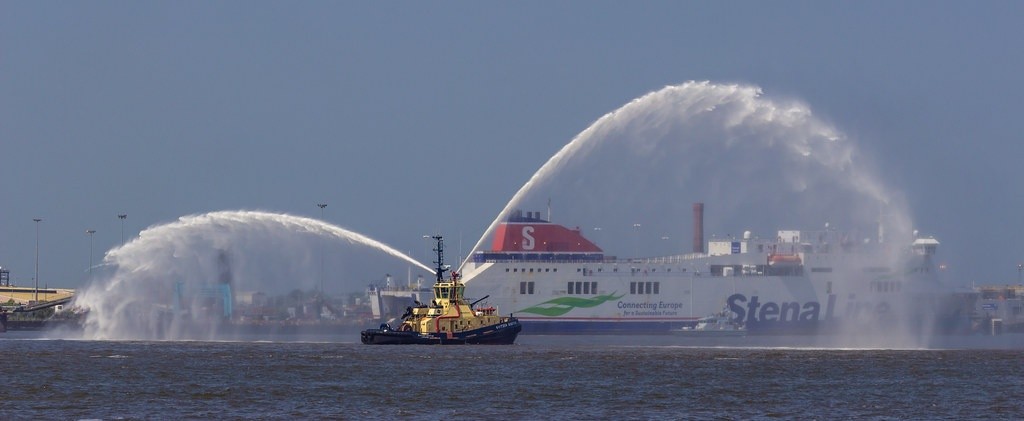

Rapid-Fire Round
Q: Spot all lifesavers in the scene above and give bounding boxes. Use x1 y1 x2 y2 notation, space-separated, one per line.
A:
464 326 468 330
452 272 455 276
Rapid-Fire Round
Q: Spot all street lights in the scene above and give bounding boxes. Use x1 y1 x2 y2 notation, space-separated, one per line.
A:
32 218 42 305
632 223 641 259
117 214 127 247
422 234 429 267
662 236 669 257
1016 264 1023 285
86 231 96 287
317 202 328 299
593 227 602 245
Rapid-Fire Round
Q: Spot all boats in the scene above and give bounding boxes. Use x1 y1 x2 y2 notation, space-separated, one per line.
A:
452 191 949 354
360 232 522 348
